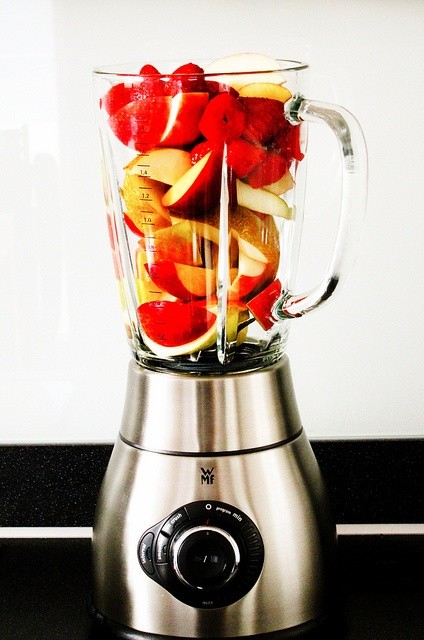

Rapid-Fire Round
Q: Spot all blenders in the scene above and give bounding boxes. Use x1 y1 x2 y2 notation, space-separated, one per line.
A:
88 55 371 639
